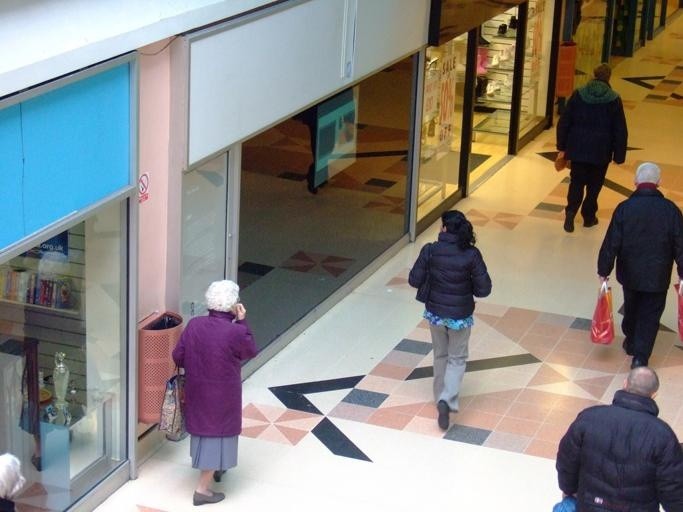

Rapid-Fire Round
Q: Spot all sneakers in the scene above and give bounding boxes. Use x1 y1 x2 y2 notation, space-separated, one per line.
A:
486 80 501 96
497 24 507 34
509 19 516 29
492 44 515 66
438 400 450 430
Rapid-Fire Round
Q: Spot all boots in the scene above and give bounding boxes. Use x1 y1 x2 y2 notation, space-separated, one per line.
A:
479 24 490 44
477 48 488 75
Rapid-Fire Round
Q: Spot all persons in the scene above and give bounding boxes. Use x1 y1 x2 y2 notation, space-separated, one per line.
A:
0 453 27 512
556 366 683 512
17 251 81 471
556 63 628 232
597 163 683 369
409 210 492 428
172 279 258 505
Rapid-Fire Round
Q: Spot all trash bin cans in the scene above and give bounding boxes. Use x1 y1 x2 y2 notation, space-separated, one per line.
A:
556 41 577 97
137 309 184 423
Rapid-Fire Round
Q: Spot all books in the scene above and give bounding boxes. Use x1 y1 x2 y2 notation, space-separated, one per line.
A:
0 273 77 307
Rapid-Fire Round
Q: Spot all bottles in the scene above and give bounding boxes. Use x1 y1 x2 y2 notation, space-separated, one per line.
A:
52 351 69 406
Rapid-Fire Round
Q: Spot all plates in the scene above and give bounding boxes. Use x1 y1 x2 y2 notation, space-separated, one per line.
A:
38 388 50 402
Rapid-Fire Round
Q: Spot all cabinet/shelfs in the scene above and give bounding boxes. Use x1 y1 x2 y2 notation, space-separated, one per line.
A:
475 31 540 135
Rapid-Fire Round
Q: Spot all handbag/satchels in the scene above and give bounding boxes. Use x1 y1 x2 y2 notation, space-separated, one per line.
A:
674 280 683 344
158 375 189 442
415 270 433 303
591 279 615 345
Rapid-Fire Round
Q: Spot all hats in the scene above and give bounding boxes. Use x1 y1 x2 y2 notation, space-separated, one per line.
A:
0 453 27 500
205 280 240 312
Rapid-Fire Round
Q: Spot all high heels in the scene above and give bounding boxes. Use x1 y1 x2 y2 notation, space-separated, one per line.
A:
213 470 227 482
194 490 225 506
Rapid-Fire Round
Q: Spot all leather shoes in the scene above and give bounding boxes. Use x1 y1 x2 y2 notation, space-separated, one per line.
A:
584 216 598 227
421 119 435 145
563 209 574 232
